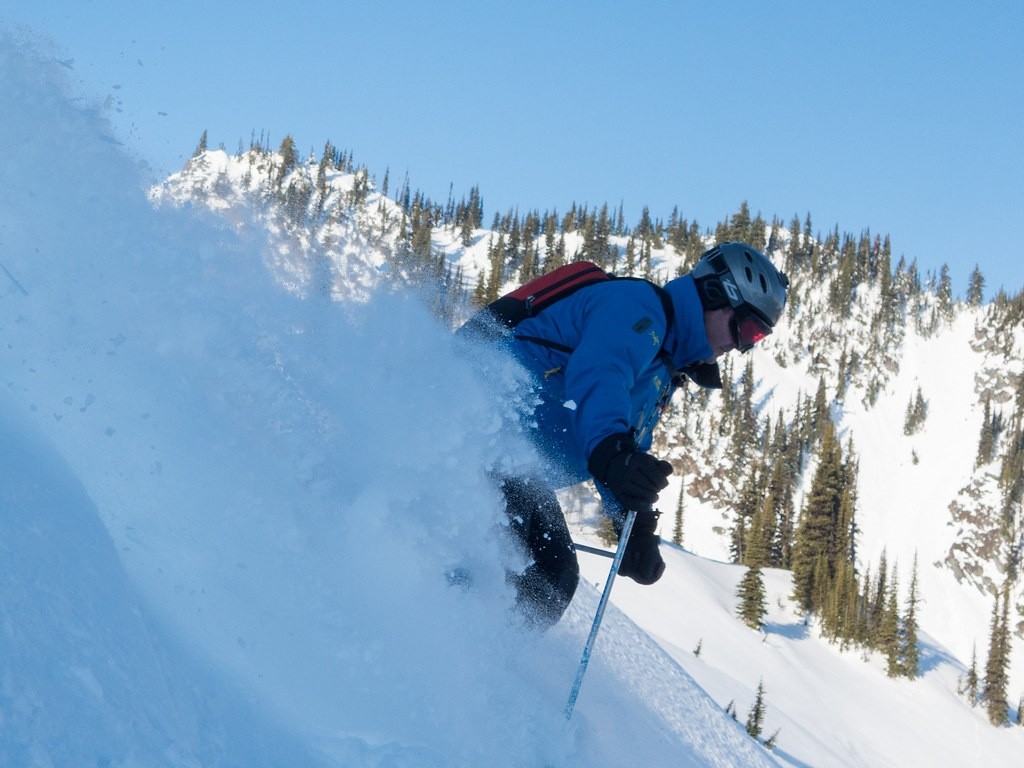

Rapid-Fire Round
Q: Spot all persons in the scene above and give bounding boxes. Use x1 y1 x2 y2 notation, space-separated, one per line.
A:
439 242 790 636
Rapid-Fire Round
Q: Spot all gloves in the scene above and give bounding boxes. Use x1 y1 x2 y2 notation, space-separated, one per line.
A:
590 432 671 514
617 526 665 586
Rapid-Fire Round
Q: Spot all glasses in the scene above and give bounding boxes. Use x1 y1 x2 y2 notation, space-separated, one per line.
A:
731 312 773 353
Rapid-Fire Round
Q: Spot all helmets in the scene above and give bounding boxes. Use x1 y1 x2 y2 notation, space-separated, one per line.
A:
690 241 788 334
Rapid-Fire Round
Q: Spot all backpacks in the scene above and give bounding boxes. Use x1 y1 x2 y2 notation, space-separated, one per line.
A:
454 259 675 365
